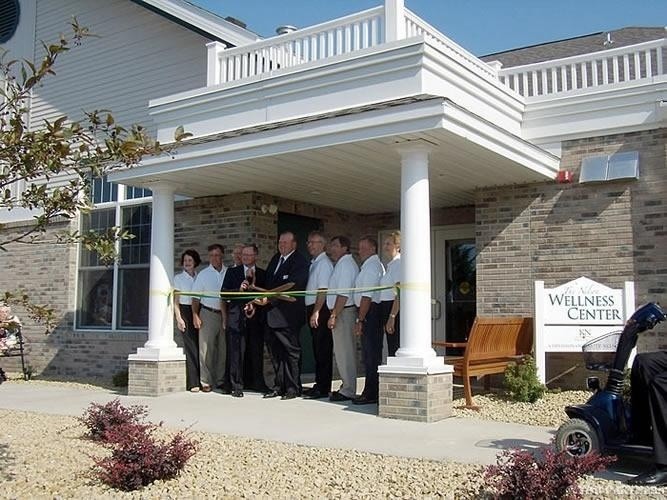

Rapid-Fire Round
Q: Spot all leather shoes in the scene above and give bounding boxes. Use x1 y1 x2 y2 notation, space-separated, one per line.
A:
190 384 377 405
627 464 666 486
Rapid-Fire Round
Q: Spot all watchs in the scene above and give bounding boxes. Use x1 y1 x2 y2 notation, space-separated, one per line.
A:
355 318 365 324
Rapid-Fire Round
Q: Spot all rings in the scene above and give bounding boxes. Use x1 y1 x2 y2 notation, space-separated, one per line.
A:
356 332 359 335
389 328 392 330
331 325 333 329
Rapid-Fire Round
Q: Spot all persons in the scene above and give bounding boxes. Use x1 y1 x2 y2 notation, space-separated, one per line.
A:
327 235 360 402
627 350 667 488
220 244 274 397
191 244 230 392
254 231 309 400
352 235 386 405
380 229 401 357
228 242 246 268
300 230 334 399
174 249 202 392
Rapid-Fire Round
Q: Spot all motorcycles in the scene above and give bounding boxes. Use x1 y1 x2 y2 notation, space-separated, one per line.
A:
553 301 667 466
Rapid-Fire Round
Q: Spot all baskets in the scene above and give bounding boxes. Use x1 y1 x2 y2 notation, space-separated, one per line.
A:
582 330 622 372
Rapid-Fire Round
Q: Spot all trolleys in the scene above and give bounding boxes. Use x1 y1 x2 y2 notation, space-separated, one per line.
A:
0 305 29 384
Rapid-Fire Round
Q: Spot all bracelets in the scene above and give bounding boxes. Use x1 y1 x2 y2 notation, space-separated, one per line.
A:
330 314 337 319
389 314 396 318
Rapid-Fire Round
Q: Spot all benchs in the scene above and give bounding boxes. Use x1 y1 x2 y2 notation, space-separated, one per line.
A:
431 316 533 410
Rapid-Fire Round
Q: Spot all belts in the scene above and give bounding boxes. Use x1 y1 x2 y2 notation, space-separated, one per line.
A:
201 305 221 314
344 305 355 308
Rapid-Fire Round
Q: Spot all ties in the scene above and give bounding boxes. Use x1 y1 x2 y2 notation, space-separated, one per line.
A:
280 257 284 266
